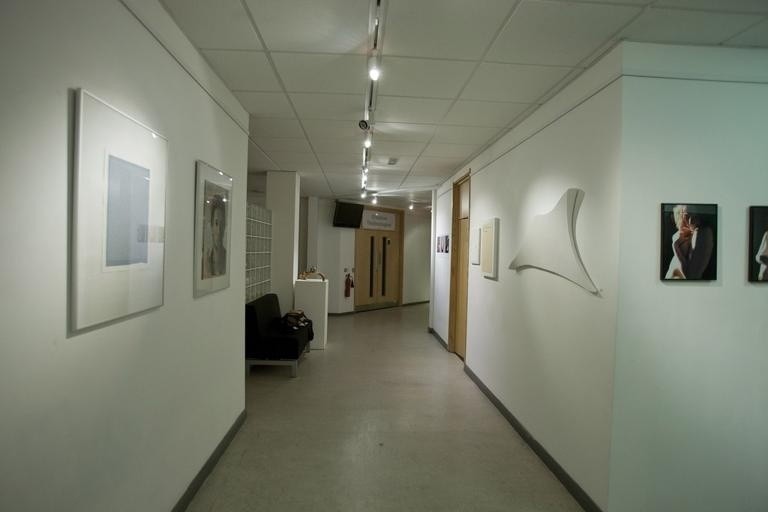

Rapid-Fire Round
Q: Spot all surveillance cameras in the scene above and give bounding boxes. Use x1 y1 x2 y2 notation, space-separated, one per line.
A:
359 119 371 131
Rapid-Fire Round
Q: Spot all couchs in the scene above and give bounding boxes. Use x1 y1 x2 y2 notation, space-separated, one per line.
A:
245 293 311 378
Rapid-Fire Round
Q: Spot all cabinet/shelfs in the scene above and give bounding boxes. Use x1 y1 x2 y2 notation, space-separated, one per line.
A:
295 278 329 350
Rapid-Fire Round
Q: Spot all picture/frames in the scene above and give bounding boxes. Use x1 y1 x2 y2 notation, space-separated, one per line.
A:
193 159 234 300
71 87 169 333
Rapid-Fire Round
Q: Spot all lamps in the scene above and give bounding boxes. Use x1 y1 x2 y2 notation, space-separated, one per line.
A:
356 16 380 192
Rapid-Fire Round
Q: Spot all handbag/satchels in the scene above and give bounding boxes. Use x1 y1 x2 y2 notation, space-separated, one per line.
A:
281 309 310 328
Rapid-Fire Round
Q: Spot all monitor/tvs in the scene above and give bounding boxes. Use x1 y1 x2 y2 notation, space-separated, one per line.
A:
333 201 364 228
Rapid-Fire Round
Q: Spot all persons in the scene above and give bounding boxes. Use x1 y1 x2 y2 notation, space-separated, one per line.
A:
664 205 718 280
202 193 227 280
